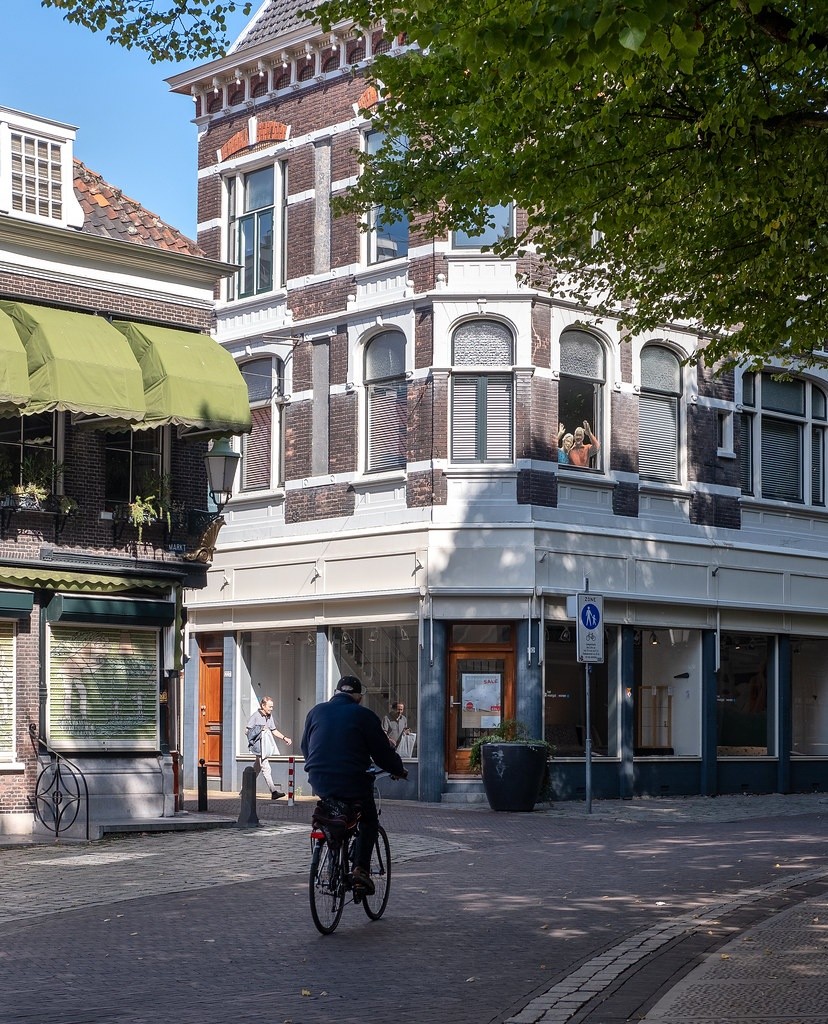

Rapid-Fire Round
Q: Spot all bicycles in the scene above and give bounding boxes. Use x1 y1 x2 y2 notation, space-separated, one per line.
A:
308 770 410 934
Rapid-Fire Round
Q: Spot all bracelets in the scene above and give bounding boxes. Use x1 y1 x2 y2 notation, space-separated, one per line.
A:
283 736 286 740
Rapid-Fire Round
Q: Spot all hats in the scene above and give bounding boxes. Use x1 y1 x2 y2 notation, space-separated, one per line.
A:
336 676 362 694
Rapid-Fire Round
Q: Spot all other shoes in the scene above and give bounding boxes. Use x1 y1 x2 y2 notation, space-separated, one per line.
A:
353 867 376 891
271 790 286 800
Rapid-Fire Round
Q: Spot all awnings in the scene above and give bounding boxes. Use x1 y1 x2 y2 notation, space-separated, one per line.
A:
0 298 253 448
0 566 182 592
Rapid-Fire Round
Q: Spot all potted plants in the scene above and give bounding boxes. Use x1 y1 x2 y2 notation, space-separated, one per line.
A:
462 722 556 813
15 458 66 510
123 501 172 533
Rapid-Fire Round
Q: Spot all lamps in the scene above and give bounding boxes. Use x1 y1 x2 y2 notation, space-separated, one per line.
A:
648 631 659 644
243 342 253 358
188 435 242 536
282 636 294 646
476 299 488 317
376 312 384 328
367 628 380 642
307 633 316 646
340 631 352 644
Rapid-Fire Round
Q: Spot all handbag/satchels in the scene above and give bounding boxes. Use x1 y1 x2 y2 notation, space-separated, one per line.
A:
395 731 417 758
261 727 280 760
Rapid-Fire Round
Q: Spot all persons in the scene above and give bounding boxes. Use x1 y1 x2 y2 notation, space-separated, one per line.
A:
301 675 409 896
381 702 410 752
238 696 292 800
558 419 600 467
